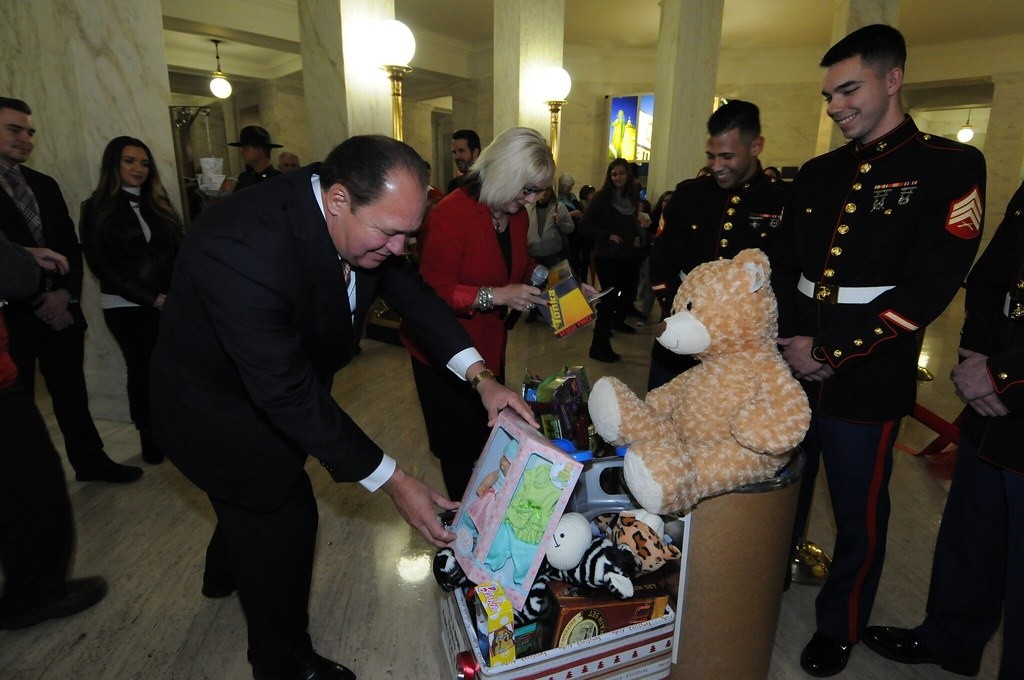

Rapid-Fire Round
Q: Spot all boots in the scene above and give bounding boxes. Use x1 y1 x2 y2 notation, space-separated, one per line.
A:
589 302 621 363
609 289 639 336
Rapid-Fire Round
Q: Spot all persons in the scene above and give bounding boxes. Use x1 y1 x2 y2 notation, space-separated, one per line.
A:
421 160 445 204
278 152 301 174
646 100 790 391
80 135 187 466
0 228 107 629
456 439 520 552
858 178 1024 680
449 129 481 173
751 23 986 678
398 125 598 501
524 157 781 364
0 96 143 481
153 135 541 680
227 126 284 192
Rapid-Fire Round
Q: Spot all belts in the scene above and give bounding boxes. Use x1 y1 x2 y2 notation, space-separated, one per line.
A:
798 270 897 306
1004 291 1024 322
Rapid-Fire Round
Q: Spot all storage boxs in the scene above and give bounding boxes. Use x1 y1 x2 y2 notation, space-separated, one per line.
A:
536 259 596 340
436 364 675 680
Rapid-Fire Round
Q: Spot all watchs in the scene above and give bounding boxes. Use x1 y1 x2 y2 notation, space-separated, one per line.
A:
472 367 496 387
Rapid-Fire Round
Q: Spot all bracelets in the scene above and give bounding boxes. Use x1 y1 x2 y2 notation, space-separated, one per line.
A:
479 285 494 313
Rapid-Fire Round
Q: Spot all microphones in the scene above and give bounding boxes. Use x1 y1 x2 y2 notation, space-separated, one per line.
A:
504 264 549 329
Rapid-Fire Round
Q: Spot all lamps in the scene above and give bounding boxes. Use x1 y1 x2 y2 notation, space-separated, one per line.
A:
956 109 974 143
536 67 571 102
209 39 232 100
368 19 416 67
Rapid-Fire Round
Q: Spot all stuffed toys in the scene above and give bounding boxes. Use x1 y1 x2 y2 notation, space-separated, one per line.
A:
586 247 812 515
432 508 682 628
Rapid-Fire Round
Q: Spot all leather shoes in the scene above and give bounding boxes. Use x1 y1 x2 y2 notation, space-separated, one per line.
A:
201 580 237 599
861 620 986 679
252 645 358 680
798 630 855 678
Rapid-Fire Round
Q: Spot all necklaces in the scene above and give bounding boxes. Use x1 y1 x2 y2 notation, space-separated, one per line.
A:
490 211 503 231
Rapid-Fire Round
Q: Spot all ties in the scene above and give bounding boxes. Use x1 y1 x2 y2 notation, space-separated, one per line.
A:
342 257 351 289
5 166 45 249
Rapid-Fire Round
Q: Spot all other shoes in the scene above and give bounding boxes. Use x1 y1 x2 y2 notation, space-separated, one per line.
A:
523 306 540 324
138 437 163 464
1 574 110 632
70 452 143 484
637 321 652 326
503 307 522 330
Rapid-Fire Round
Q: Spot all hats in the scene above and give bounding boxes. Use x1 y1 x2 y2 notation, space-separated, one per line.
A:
226 125 284 149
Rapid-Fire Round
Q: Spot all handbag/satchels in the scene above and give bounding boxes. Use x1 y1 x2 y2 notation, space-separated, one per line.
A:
562 241 574 262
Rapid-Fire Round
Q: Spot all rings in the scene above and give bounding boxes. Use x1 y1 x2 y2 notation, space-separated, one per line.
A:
527 304 532 309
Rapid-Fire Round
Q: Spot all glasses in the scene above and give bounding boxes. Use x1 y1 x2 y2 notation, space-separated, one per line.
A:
521 186 546 198
